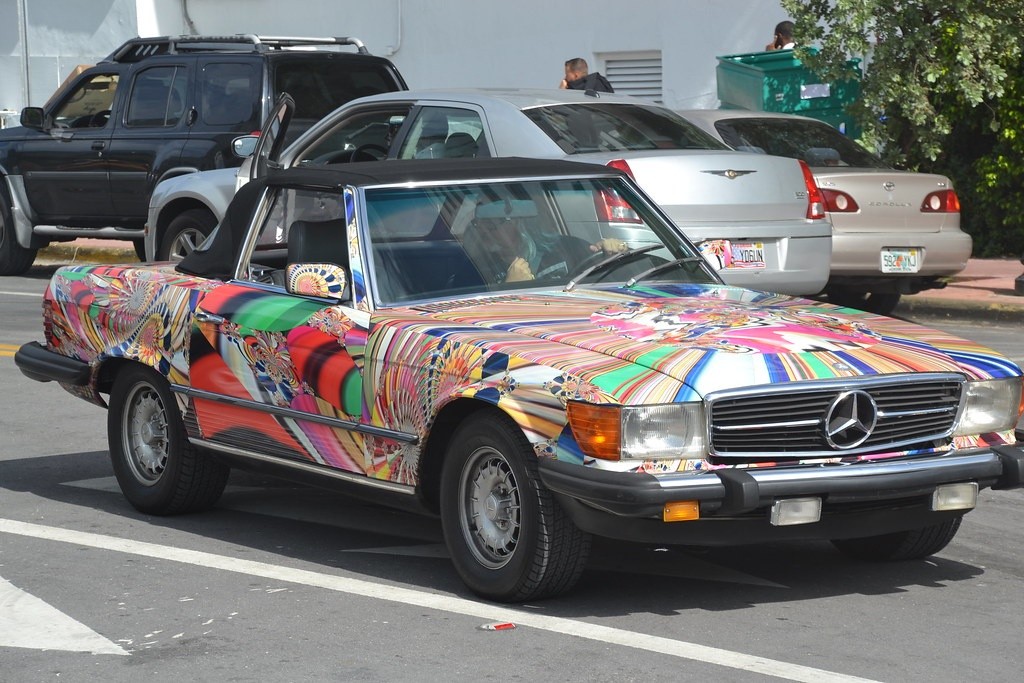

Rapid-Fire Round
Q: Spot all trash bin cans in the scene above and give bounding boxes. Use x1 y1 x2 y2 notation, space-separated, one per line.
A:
715 51 862 140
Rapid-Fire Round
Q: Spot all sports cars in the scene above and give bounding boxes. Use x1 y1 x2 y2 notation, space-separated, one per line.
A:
10 155 1023 609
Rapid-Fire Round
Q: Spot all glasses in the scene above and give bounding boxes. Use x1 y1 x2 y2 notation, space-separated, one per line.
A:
471 217 511 229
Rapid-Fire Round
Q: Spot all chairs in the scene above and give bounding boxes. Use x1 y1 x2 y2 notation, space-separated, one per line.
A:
442 207 568 295
268 217 408 304
805 148 840 165
136 85 181 126
443 132 479 159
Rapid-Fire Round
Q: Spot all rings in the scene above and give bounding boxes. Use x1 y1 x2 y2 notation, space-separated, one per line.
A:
620 243 624 247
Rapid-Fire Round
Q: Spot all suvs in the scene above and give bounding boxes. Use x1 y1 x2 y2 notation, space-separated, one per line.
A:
0 31 411 273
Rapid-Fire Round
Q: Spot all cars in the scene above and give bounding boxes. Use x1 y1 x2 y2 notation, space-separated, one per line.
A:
656 106 974 317
141 87 834 301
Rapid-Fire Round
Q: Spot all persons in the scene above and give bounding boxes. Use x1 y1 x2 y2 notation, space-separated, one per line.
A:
451 198 632 286
554 58 614 93
764 22 804 52
